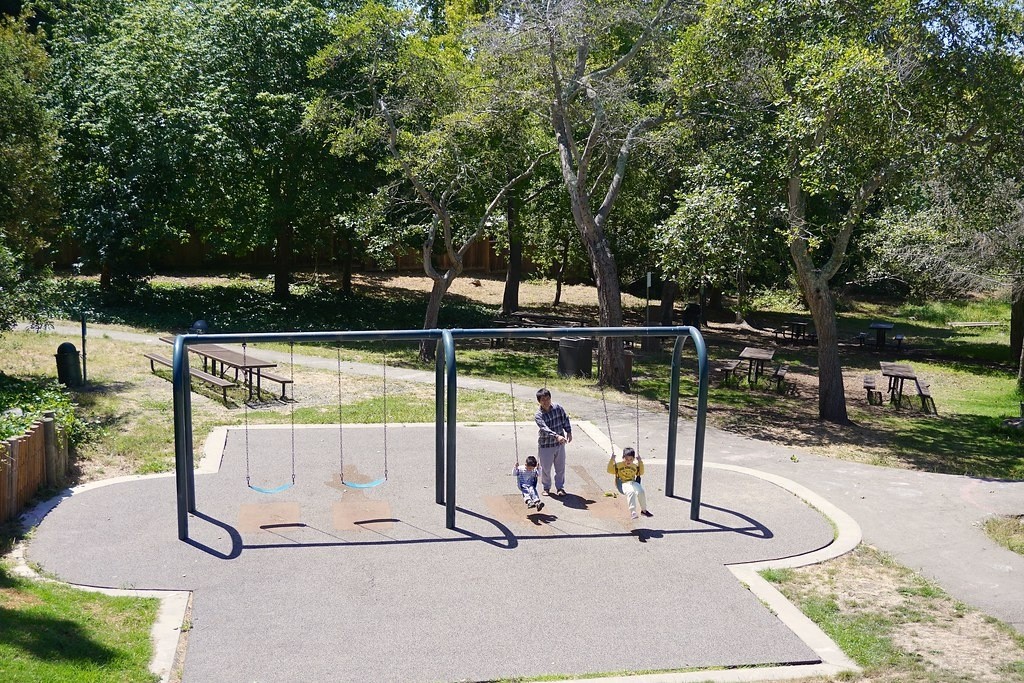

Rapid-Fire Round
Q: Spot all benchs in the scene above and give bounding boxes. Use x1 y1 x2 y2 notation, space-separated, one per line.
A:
511 311 593 327
144 353 238 405
772 364 791 385
894 332 904 348
721 359 742 380
916 379 930 408
857 332 869 346
863 374 876 402
772 326 790 340
809 331 817 344
220 362 294 400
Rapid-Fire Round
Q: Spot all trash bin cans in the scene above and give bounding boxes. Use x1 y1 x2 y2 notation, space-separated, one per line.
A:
54 342 82 387
557 336 592 377
683 303 701 330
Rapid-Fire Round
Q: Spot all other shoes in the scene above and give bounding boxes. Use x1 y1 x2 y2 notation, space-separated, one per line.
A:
542 489 550 495
632 512 638 519
557 489 566 496
528 503 535 508
642 510 653 516
536 503 544 511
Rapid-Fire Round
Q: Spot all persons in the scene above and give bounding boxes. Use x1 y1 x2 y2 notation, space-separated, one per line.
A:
513 457 545 512
535 389 573 497
607 448 653 518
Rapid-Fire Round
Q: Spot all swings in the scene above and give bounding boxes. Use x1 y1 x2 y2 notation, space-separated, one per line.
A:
334 338 389 490
503 335 553 492
589 335 642 495
240 341 296 494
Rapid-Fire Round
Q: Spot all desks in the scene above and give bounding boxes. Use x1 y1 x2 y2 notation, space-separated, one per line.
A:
494 320 595 350
788 322 808 341
159 337 277 404
879 361 917 410
869 322 894 348
738 347 778 382
